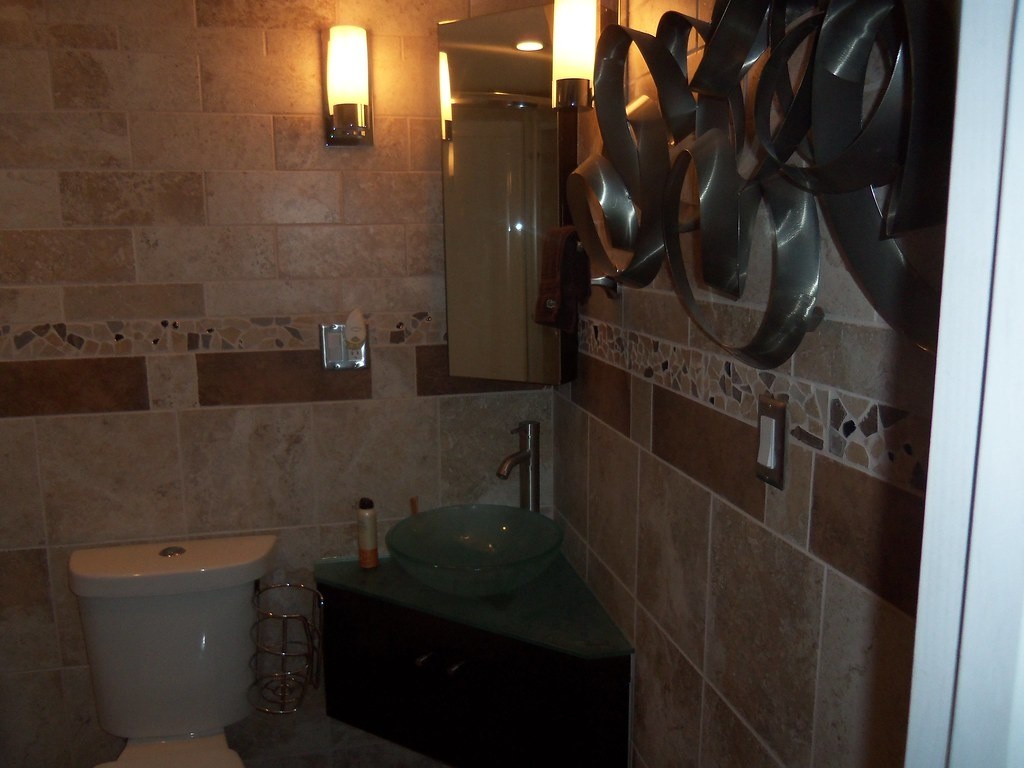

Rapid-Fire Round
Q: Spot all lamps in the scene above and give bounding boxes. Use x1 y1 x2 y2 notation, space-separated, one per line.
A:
553 0 634 112
326 25 375 149
438 51 453 143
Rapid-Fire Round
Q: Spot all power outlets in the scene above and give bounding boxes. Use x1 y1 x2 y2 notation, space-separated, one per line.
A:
320 323 368 371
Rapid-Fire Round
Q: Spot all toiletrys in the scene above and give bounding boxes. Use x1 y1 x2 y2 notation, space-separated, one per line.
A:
356 496 379 570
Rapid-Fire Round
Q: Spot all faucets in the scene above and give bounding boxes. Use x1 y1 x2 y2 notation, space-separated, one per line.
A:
495 419 541 514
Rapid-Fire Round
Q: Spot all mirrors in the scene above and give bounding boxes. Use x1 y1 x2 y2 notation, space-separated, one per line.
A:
437 2 578 385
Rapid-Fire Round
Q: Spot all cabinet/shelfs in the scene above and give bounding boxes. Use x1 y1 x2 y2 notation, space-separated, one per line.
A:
316 582 631 768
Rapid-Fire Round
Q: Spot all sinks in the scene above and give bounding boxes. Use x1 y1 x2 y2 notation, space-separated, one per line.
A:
384 502 565 598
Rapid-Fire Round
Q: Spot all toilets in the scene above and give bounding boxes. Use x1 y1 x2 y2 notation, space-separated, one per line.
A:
64 531 280 768
